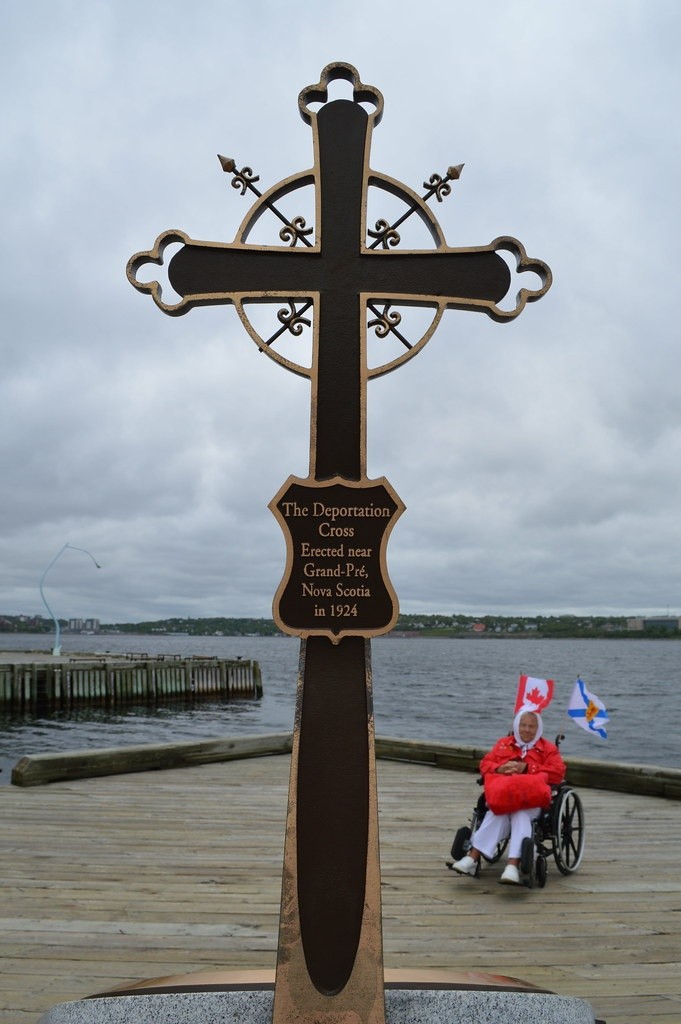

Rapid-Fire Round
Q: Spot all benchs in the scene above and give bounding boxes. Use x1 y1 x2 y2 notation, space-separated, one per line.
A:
69 652 217 662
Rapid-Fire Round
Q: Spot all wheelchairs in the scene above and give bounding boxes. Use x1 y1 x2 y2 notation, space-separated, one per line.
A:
444 734 586 891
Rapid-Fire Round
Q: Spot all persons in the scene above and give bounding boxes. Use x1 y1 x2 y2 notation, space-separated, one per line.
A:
452 710 566 883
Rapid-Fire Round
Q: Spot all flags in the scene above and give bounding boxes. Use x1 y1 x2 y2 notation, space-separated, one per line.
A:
566 679 609 740
513 675 554 715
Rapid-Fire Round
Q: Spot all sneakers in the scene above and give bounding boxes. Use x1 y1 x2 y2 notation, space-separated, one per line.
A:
452 857 478 877
502 866 519 883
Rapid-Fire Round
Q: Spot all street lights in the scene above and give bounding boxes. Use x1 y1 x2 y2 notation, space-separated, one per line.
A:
38 543 102 658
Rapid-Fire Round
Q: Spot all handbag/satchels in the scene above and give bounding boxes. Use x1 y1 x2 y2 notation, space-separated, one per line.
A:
485 772 553 816
